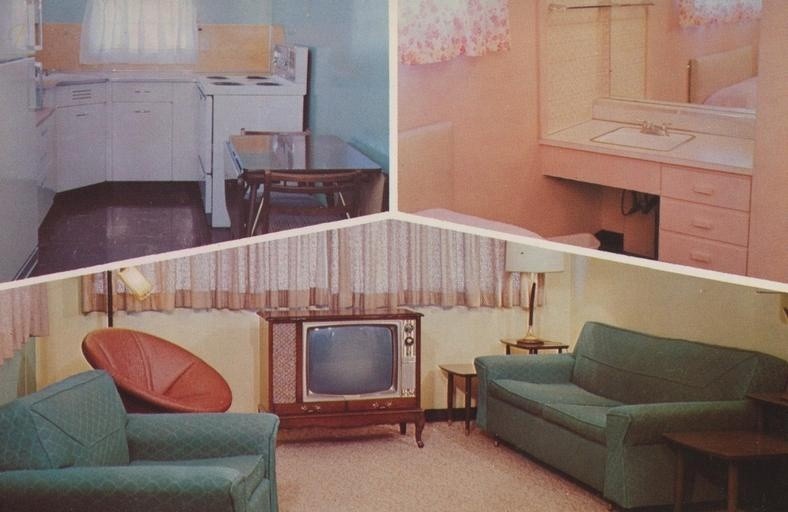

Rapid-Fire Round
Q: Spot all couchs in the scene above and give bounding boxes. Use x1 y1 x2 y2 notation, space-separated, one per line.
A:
473 326 787 512
80 328 240 415
2 374 280 508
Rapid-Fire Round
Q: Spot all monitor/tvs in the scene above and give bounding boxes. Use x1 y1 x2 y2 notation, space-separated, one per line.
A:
303 320 415 402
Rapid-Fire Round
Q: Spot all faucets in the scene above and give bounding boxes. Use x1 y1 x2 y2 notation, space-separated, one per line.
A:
35 60 50 77
640 119 670 138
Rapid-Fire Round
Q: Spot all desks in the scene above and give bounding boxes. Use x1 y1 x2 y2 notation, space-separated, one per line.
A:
659 389 787 512
221 129 379 236
501 340 568 356
438 362 482 426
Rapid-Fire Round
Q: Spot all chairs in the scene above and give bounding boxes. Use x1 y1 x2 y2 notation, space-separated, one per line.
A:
253 166 362 236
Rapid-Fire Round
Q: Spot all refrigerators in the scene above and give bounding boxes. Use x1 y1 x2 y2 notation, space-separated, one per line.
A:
0 0 44 285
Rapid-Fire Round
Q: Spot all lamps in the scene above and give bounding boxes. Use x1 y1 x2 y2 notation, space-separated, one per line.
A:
104 266 153 327
502 242 563 343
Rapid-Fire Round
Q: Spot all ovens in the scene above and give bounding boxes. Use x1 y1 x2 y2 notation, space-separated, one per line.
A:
190 89 214 221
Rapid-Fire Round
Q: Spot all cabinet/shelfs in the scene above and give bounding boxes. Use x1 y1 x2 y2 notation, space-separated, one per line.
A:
37 110 58 224
253 307 427 450
109 80 172 183
51 84 107 199
657 160 749 274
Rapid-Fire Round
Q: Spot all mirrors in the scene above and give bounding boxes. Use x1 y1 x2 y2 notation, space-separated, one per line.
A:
598 5 762 118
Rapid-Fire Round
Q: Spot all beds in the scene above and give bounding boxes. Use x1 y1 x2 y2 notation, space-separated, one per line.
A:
683 48 762 111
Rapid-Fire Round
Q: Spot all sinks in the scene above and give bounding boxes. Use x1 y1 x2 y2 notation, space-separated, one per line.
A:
591 126 695 153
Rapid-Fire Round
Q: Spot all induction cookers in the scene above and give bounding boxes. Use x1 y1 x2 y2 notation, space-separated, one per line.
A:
193 72 306 97
